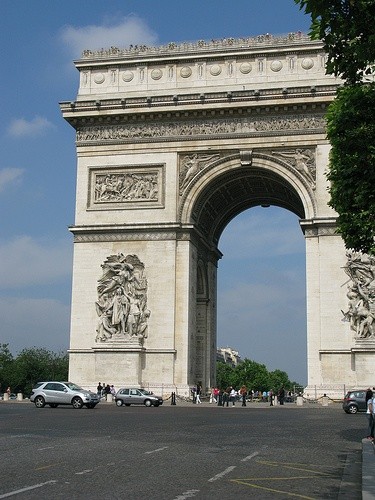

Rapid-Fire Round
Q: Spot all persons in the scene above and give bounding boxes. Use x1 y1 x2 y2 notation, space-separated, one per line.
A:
95 264 150 340
256 388 273 406
7 387 10 399
366 390 375 440
95 174 156 201
278 149 315 186
180 154 214 191
280 386 284 405
240 386 247 406
346 252 375 338
97 382 116 401
209 386 236 407
196 384 201 404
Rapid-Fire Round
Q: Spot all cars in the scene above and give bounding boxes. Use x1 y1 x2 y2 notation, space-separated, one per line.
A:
114 387 164 408
342 390 375 414
30 381 99 409
